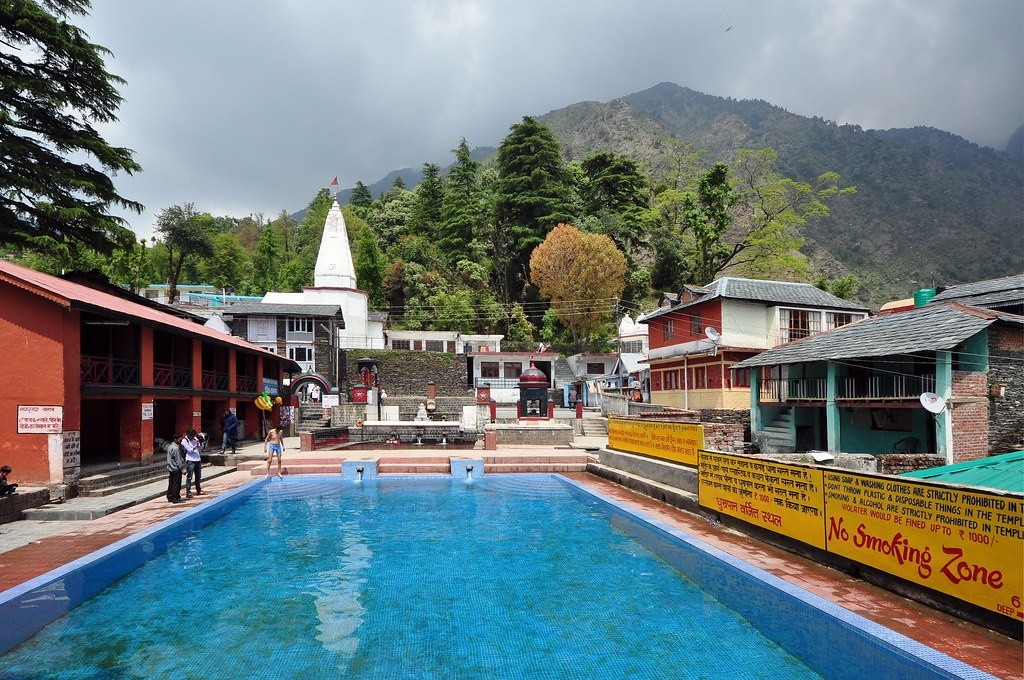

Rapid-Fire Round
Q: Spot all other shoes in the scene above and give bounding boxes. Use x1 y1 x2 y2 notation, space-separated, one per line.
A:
196 490 208 495
186 491 193 498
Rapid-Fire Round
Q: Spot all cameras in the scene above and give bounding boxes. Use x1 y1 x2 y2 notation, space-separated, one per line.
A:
197 435 204 442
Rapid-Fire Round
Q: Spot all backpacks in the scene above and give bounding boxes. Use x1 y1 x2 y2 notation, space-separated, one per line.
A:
179 444 188 460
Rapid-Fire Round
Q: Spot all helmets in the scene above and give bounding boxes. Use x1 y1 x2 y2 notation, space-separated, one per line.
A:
197 432 208 443
